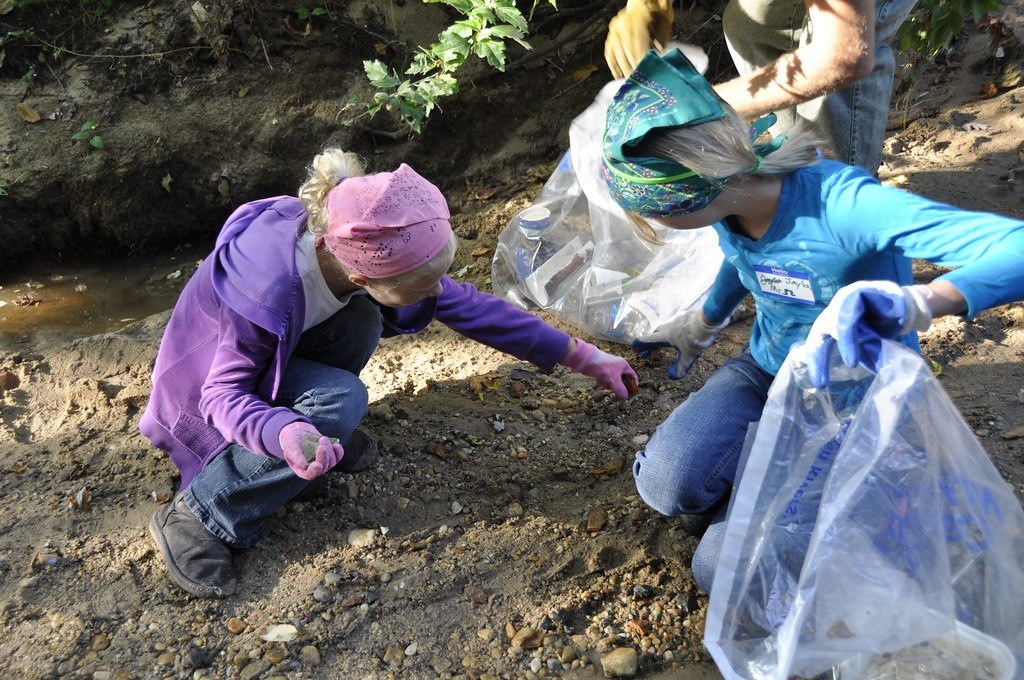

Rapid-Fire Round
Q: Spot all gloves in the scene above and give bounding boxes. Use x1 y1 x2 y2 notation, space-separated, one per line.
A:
806 277 931 390
558 336 640 400
278 421 345 479
632 307 731 380
604 0 677 80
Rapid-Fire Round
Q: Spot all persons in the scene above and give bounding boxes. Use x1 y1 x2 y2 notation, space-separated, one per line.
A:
602 45 1024 644
605 0 915 177
140 148 639 600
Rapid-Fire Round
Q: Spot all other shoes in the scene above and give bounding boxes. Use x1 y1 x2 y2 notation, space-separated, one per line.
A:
329 426 376 472
148 494 239 597
